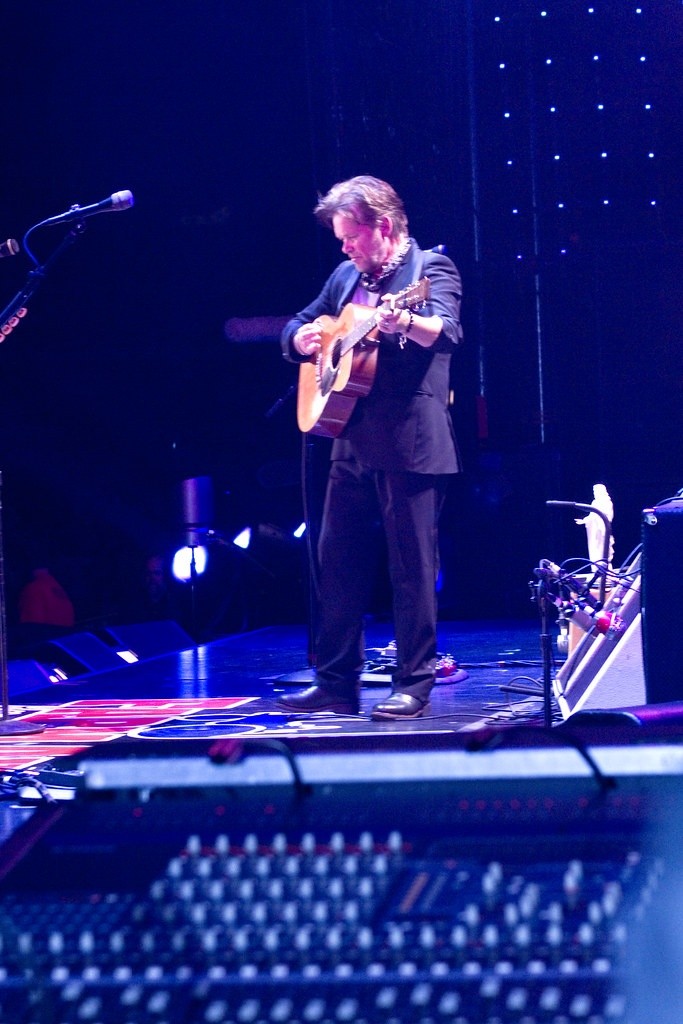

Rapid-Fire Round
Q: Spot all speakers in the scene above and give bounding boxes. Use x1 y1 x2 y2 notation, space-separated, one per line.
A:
550 553 647 721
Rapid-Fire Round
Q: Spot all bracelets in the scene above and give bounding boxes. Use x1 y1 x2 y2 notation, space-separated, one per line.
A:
401 309 414 335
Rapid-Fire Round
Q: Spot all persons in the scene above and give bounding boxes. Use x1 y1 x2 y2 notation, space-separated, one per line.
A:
276 165 469 723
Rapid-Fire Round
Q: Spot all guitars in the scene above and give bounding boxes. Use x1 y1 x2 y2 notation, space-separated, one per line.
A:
295 275 432 441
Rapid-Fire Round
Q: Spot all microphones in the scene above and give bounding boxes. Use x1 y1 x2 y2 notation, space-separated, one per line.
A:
0 238 20 258
39 189 135 226
544 560 603 612
422 245 447 256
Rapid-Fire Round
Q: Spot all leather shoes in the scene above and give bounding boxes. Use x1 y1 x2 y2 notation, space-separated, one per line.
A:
274 685 360 716
370 692 432 720
